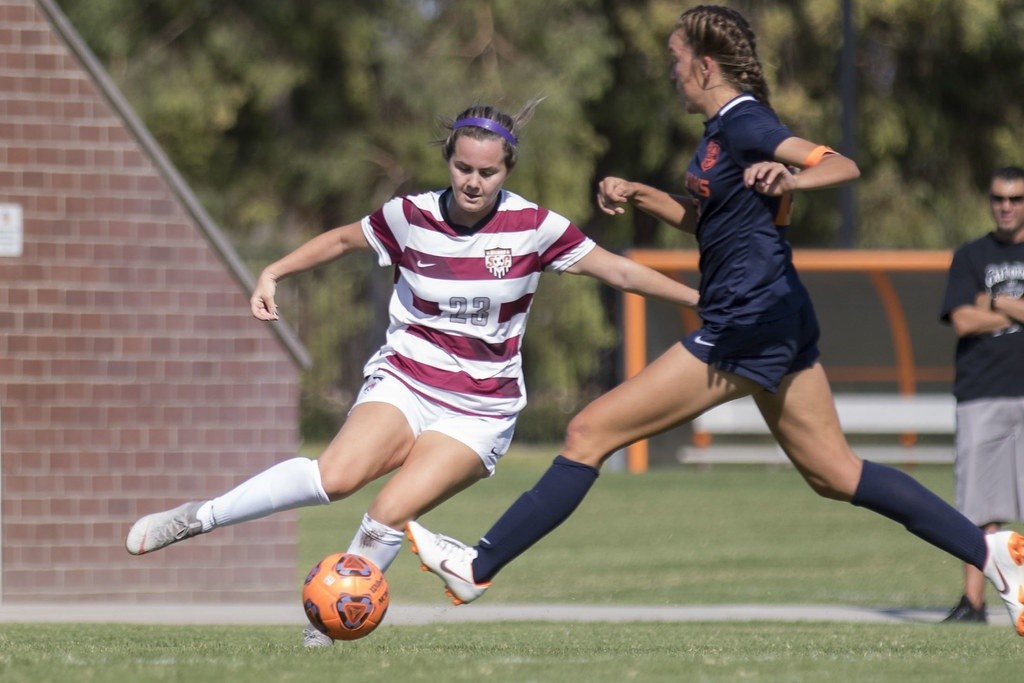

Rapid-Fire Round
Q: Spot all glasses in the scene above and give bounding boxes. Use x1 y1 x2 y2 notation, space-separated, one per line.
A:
991 196 1024 209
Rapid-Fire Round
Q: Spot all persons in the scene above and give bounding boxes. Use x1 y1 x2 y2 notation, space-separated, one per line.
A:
124 91 699 644
936 164 1024 622
402 5 1024 638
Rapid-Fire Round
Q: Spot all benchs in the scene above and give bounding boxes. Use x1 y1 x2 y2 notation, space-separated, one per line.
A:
675 394 957 471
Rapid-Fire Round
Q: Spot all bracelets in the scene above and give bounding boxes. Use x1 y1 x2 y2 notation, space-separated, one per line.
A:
989 293 998 311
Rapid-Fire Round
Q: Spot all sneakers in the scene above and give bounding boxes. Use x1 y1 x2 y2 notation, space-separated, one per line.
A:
303 623 334 648
983 531 1024 636
406 521 493 605
941 595 986 623
125 502 203 555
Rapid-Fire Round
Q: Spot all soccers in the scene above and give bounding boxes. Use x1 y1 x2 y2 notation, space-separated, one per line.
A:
299 549 394 643
